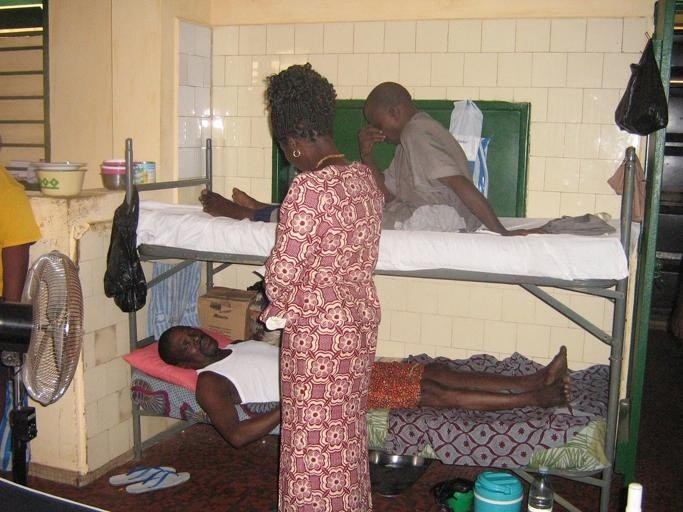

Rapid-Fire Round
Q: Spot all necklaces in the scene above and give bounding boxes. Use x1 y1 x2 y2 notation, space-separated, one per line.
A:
315 154 347 168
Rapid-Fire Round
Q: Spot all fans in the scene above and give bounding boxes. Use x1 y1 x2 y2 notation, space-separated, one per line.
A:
0 250 86 487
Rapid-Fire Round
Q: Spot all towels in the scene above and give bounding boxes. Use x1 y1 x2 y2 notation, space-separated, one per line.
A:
147 261 202 341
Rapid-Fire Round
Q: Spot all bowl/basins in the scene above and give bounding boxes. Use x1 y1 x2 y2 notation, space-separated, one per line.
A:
1 161 137 197
364 451 432 498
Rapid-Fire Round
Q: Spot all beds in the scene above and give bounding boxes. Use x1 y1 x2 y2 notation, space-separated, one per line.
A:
121 133 637 511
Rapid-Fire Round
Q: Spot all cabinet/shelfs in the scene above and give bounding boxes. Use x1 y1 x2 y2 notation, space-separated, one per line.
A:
626 2 683 512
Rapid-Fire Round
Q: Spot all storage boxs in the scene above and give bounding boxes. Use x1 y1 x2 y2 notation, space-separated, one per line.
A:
195 282 256 342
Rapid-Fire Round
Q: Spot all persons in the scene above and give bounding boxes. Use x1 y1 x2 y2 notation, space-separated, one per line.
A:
0 163 40 483
197 82 548 236
257 63 383 511
158 325 569 450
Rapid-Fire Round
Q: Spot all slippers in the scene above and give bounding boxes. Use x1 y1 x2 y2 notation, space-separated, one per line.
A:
108 466 177 487
126 471 190 495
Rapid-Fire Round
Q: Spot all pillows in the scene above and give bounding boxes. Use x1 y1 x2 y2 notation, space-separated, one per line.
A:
123 325 231 392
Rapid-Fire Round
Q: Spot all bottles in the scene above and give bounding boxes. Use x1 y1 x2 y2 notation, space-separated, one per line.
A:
526 464 555 512
